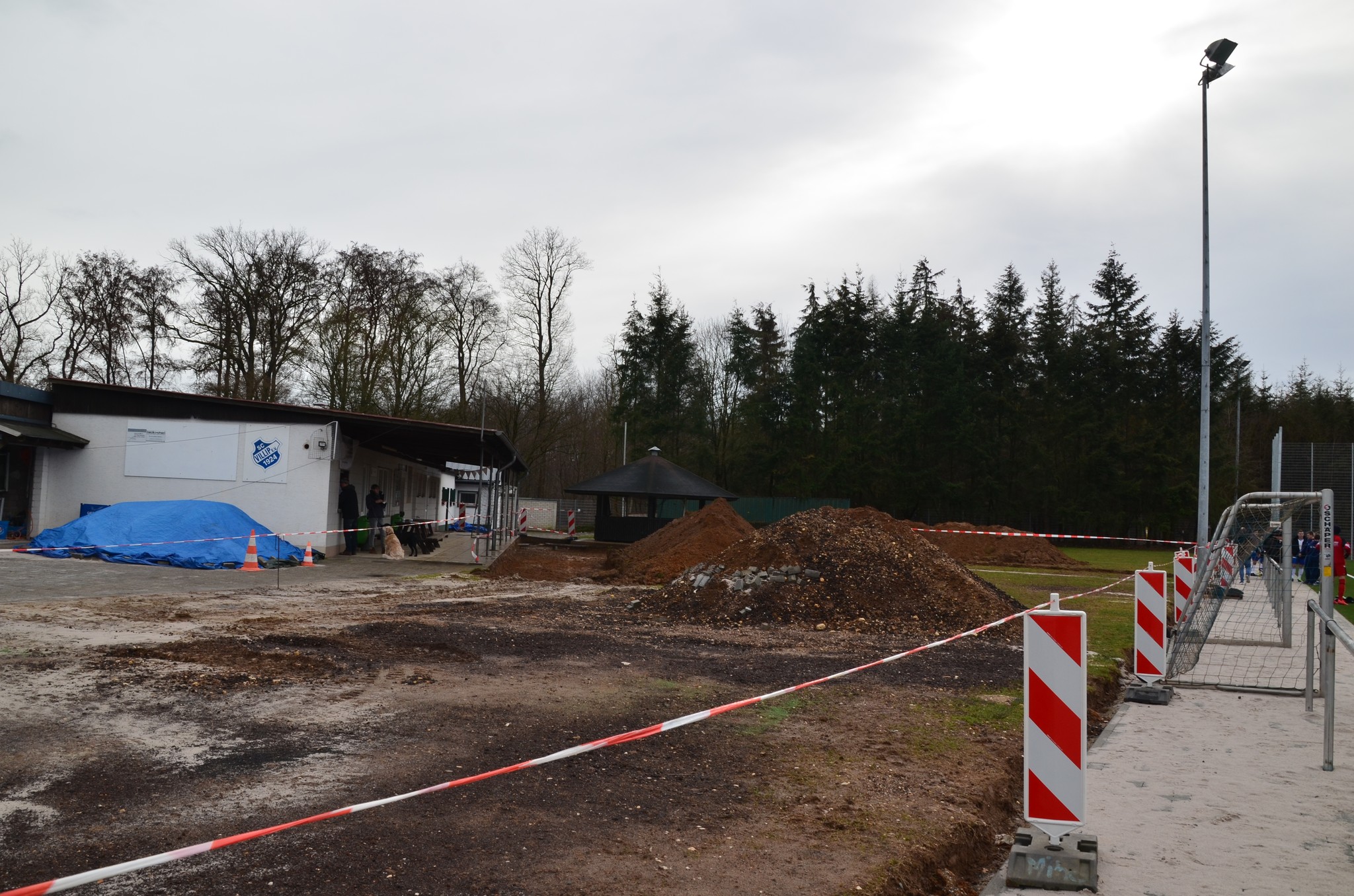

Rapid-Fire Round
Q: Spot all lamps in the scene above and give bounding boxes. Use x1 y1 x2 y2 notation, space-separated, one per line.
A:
318 441 328 451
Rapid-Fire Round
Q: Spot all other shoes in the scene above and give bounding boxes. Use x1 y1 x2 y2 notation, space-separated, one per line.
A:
1291 578 1294 581
1310 583 1315 585
339 550 352 555
1298 578 1301 582
1239 581 1244 584
351 549 357 555
1338 599 1348 605
1334 600 1338 604
1303 580 1310 584
1245 574 1250 583
1250 573 1258 577
1259 568 1263 571
369 547 377 554
382 547 386 554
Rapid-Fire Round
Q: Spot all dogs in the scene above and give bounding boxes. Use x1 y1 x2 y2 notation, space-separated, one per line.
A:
380 523 422 557
381 525 405 561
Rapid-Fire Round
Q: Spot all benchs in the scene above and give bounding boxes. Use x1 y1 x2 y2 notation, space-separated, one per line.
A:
425 531 451 542
374 533 382 538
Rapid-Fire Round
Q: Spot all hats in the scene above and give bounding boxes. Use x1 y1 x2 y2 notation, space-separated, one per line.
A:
1273 531 1282 536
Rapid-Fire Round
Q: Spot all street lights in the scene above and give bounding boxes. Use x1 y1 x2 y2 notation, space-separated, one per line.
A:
1196 37 1238 588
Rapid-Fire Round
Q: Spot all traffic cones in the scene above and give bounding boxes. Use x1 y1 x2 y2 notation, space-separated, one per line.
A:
301 541 315 567
235 529 267 571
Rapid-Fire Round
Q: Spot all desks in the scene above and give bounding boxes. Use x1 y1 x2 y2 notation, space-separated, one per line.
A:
377 518 449 555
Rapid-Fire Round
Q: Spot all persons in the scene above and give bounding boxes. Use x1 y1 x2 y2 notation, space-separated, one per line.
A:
365 484 387 554
1291 530 1305 581
1299 530 1315 584
338 477 359 556
1234 528 1251 584
1249 526 1282 576
1306 534 1320 585
1316 525 1351 605
